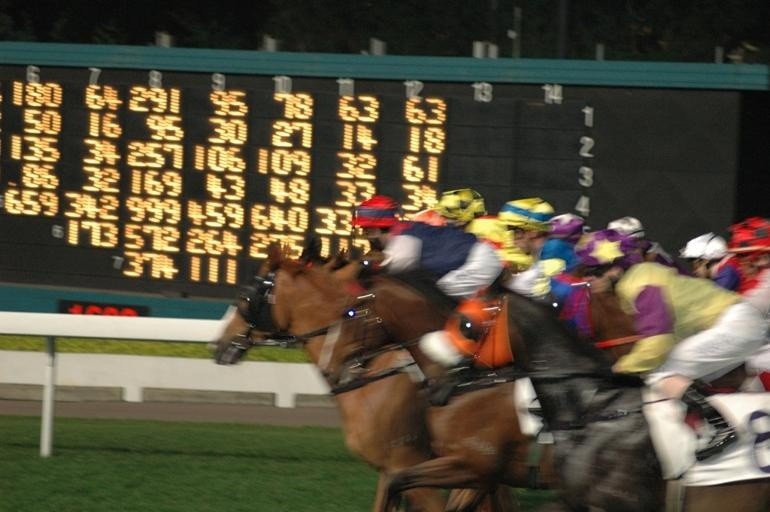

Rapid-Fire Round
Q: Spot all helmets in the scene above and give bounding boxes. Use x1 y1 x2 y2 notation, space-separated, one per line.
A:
350 195 406 226
680 234 730 262
432 187 488 219
726 217 768 264
498 197 646 275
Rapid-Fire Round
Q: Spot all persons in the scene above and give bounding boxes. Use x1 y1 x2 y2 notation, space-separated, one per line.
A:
355 187 769 482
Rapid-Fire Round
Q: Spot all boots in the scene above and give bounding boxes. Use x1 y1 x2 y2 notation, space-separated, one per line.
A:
683 384 736 459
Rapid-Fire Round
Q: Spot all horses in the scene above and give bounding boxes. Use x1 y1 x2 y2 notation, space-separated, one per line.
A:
207 239 770 512
